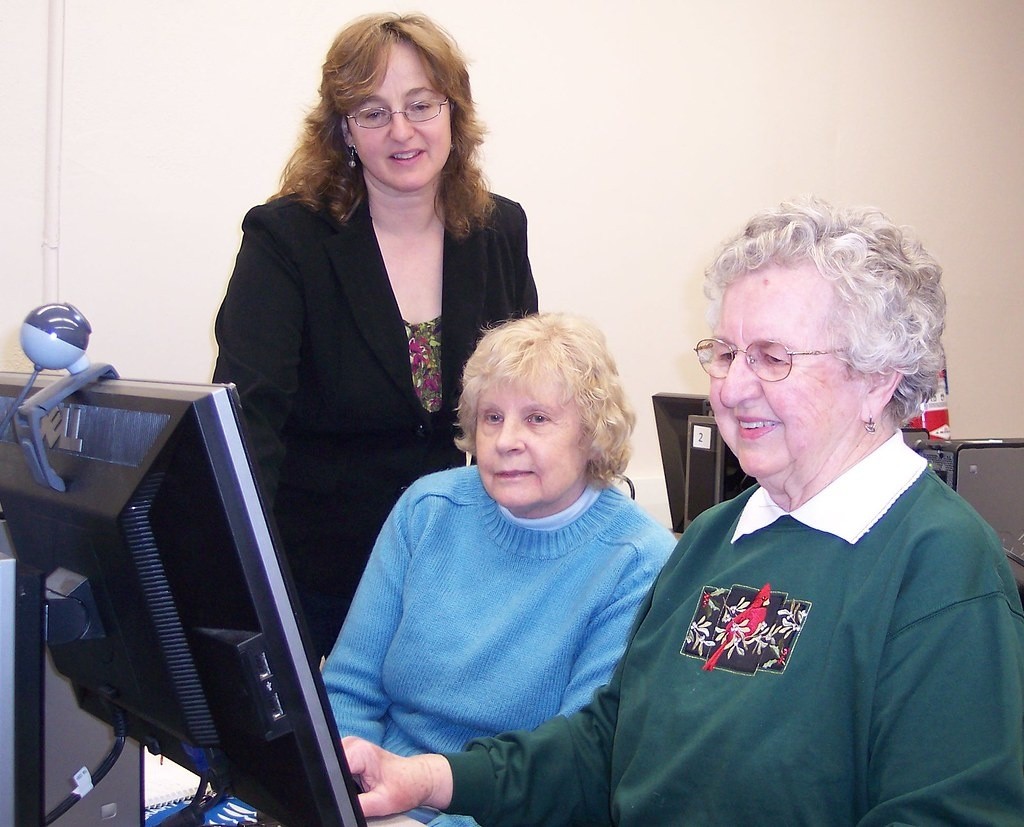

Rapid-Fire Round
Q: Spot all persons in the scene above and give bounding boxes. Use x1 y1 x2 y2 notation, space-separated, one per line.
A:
317 309 678 827
340 200 1024 827
207 13 538 670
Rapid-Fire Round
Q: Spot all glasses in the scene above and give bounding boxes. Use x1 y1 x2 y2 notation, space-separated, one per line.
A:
694 338 848 381
345 98 449 129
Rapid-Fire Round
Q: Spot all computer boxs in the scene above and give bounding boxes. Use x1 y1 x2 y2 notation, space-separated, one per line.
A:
913 439 1024 601
43 653 145 827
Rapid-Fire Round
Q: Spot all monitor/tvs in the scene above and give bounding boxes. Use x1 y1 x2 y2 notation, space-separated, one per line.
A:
652 392 756 533
0 372 367 827
